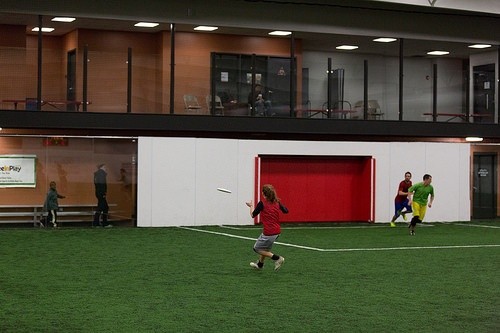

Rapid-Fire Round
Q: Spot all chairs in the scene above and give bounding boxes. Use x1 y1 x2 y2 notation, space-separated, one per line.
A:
206 95 224 115
183 95 203 114
369 100 384 120
248 103 267 117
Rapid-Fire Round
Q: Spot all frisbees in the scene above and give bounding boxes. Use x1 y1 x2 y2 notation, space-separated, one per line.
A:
217 188 232 193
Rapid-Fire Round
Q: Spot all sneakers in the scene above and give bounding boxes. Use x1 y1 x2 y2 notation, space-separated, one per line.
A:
250 262 264 270
275 255 285 271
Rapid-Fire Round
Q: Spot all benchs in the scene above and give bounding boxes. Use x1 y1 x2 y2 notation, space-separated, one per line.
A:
0 203 119 227
423 112 492 123
281 108 357 119
2 99 91 111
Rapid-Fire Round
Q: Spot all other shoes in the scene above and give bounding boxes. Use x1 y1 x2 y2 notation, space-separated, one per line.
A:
409 230 415 235
92 225 100 229
391 221 395 227
105 225 113 228
400 212 407 220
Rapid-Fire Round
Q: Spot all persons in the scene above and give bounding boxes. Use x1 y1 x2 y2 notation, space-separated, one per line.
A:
40 182 66 229
390 172 413 227
248 84 275 116
57 165 67 187
91 164 113 229
118 168 132 194
407 174 434 235
246 184 289 271
